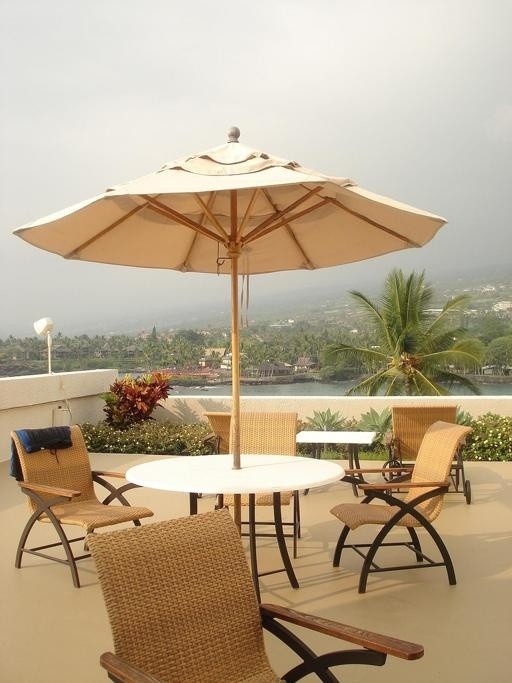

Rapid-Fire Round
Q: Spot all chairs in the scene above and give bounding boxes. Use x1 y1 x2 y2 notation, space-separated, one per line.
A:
199 414 231 454
83 506 425 682
380 404 471 504
215 412 303 559
10 424 154 592
328 420 474 595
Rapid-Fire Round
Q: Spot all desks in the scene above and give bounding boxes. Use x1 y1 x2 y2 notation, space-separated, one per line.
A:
295 430 377 497
124 452 346 602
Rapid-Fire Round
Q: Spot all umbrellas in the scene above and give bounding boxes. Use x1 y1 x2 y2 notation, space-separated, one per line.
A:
11 126 448 470
